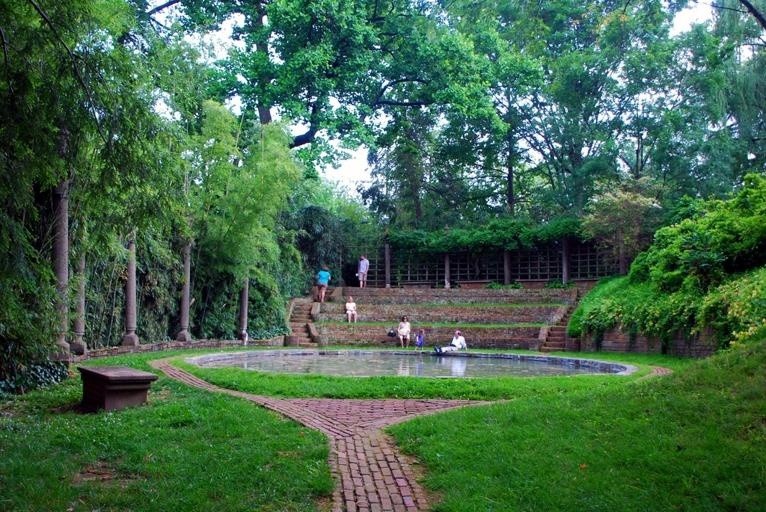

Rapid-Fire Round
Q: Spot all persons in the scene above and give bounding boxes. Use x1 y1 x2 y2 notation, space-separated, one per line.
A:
433 356 465 377
416 330 419 342
345 296 358 323
432 330 467 354
398 355 409 375
414 328 423 350
315 265 331 302
397 316 411 348
357 255 369 288
415 355 424 375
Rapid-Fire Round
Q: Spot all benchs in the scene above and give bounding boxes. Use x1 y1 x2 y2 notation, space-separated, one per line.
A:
75 366 160 414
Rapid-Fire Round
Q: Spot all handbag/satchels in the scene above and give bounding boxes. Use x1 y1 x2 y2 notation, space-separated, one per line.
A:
388 326 396 337
